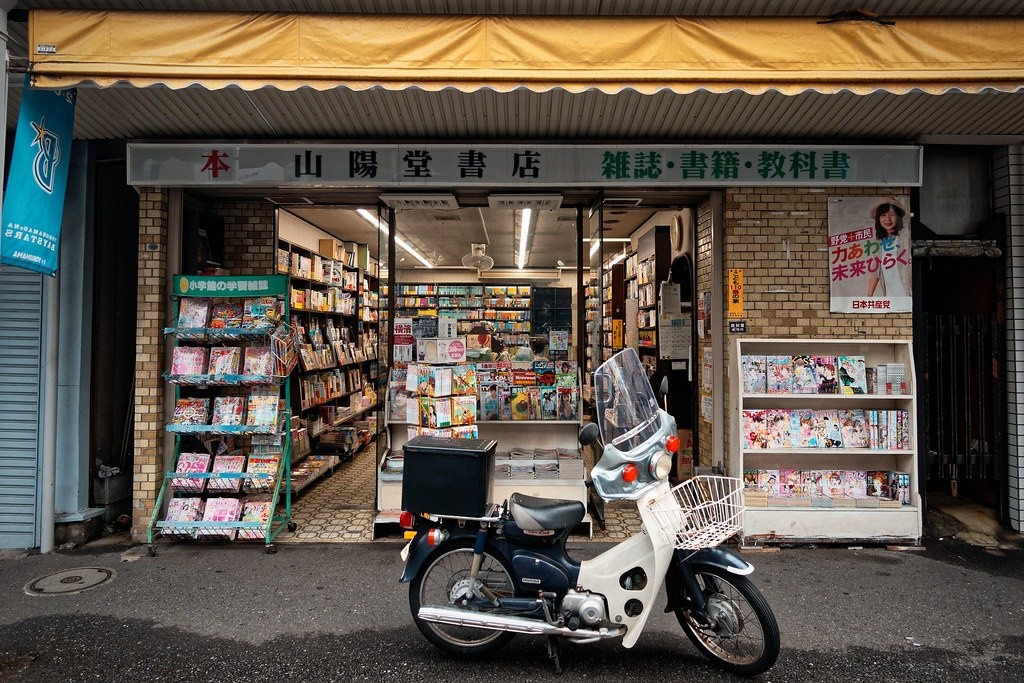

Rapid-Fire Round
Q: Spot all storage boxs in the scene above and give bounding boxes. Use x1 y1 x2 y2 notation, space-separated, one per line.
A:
420 398 451 428
421 429 452 438
419 366 452 397
451 395 477 425
417 340 467 363
319 238 338 260
451 425 478 440
452 365 477 394
311 255 321 281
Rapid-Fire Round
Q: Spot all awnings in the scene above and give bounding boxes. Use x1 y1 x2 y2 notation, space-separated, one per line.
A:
29 5 1024 97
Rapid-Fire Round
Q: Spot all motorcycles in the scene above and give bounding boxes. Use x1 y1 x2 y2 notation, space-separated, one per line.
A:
397 345 783 679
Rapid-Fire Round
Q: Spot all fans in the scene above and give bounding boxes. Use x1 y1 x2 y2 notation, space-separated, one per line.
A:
461 244 495 271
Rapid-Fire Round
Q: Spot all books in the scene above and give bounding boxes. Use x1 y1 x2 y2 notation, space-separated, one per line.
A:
162 240 912 544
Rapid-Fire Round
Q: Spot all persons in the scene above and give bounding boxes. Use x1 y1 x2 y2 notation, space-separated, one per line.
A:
428 406 436 425
561 362 569 374
543 389 575 420
484 384 499 419
391 384 411 420
867 199 913 297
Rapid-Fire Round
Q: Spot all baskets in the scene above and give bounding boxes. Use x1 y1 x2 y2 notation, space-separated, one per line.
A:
645 475 745 550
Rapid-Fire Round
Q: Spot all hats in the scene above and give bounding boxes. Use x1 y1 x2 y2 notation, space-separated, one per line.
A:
870 196 907 219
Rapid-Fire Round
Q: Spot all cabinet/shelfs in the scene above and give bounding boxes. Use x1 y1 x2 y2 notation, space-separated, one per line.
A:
145 225 694 558
727 337 924 549
